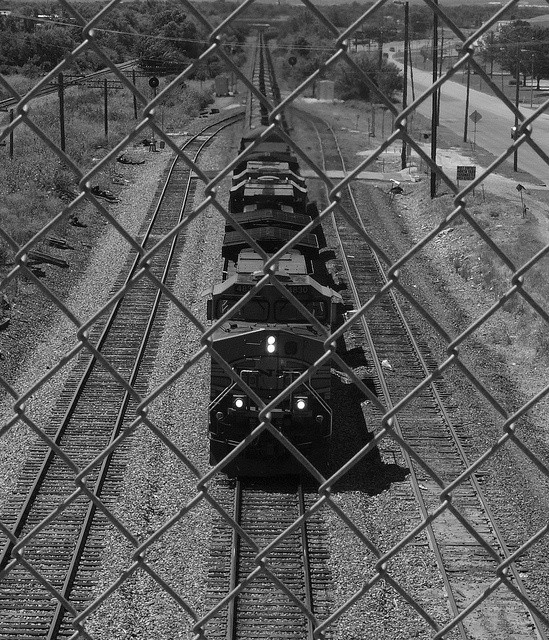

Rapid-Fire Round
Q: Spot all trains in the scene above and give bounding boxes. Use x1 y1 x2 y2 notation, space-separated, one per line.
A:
206 27 344 479
